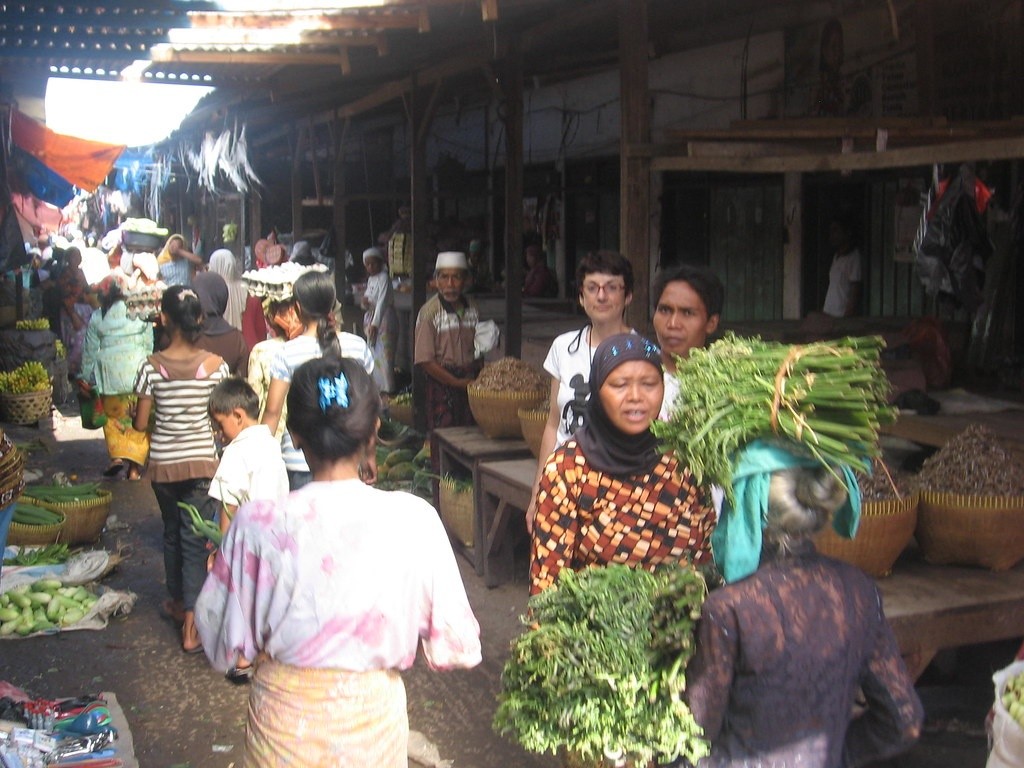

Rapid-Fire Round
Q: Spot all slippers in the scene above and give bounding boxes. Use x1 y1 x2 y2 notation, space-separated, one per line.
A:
228 665 252 680
127 473 141 482
103 462 123 477
187 643 204 653
159 599 184 628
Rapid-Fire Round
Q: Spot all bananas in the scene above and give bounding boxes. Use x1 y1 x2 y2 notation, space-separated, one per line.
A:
0 360 51 394
16 317 50 330
55 339 64 357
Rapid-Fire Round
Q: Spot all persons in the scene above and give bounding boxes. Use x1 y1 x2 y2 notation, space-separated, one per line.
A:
527 333 725 634
413 252 500 516
466 239 484 289
823 218 864 321
650 442 924 768
525 250 660 538
652 266 726 523
522 246 546 298
379 195 411 378
207 378 290 683
193 356 481 768
20 216 410 655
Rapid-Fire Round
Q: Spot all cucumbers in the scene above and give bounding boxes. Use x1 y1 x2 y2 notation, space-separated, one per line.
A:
23 481 101 502
11 503 63 525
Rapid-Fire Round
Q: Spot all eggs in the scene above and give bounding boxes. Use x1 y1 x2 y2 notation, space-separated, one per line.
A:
128 291 163 312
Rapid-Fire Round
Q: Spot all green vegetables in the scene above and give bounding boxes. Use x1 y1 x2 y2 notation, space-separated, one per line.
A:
490 559 711 767
648 339 897 506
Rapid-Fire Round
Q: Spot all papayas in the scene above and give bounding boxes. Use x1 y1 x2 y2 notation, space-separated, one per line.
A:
373 449 417 485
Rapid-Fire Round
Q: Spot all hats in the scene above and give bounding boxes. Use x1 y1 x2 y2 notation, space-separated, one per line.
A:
436 251 468 270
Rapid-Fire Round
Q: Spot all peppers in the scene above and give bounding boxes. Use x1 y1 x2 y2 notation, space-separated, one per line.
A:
0 542 85 567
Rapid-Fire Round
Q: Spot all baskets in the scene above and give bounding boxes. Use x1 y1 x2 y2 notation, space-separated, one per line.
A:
0 385 53 426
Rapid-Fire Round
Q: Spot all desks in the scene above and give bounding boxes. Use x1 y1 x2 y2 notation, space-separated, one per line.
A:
865 563 1024 687
429 423 529 575
477 460 543 586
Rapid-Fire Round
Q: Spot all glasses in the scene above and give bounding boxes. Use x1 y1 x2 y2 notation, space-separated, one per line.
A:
583 280 626 295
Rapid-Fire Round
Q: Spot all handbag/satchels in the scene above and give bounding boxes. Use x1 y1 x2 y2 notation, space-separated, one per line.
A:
77 381 109 429
